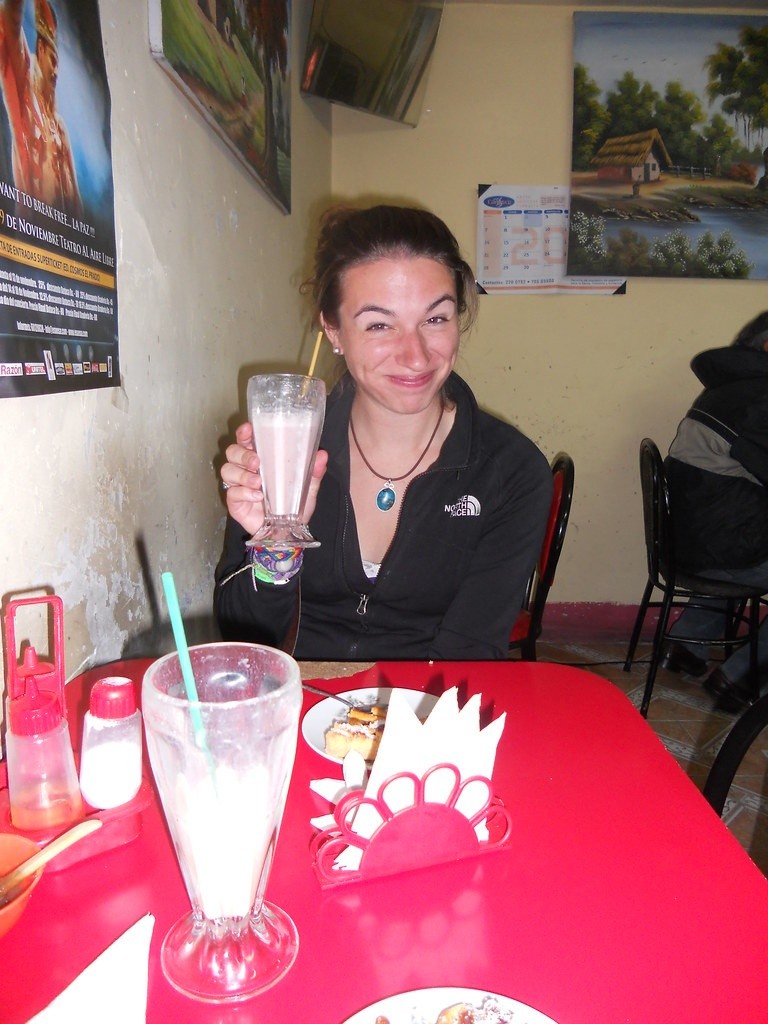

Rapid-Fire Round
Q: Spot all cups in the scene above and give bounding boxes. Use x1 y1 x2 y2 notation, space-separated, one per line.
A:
244 373 326 548
141 642 303 1004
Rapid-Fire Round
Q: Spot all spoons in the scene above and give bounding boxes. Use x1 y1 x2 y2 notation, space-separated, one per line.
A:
301 683 388 714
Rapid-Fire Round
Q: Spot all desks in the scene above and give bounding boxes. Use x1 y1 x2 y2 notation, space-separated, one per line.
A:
0 658 768 1024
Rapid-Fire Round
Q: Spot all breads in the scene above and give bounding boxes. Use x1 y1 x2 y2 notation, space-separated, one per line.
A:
324 707 390 760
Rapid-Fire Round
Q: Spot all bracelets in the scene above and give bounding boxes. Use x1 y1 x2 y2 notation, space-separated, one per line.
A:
248 546 303 584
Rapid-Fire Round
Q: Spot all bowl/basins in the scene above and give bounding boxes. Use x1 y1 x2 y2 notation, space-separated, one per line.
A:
0 832 44 938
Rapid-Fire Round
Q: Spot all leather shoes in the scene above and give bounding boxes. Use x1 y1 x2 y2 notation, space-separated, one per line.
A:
703 668 759 712
659 638 707 676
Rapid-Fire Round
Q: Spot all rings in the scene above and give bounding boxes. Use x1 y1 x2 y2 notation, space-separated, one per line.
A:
222 481 229 490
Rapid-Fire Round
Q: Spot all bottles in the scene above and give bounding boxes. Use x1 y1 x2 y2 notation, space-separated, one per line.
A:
80 677 142 810
4 647 81 830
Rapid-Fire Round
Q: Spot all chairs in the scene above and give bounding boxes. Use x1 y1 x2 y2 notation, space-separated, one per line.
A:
507 451 575 662
624 438 768 719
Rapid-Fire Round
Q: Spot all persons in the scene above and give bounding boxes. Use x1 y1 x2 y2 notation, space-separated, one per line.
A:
213 204 553 659
660 312 768 707
0 0 89 232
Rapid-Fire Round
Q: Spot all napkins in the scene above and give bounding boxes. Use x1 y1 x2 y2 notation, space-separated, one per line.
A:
310 687 507 872
26 912 155 1024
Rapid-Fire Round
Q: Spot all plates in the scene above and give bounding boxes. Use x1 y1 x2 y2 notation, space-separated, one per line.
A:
341 987 558 1024
302 686 439 771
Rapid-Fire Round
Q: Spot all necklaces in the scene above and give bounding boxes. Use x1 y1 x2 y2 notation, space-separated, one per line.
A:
349 401 444 512
